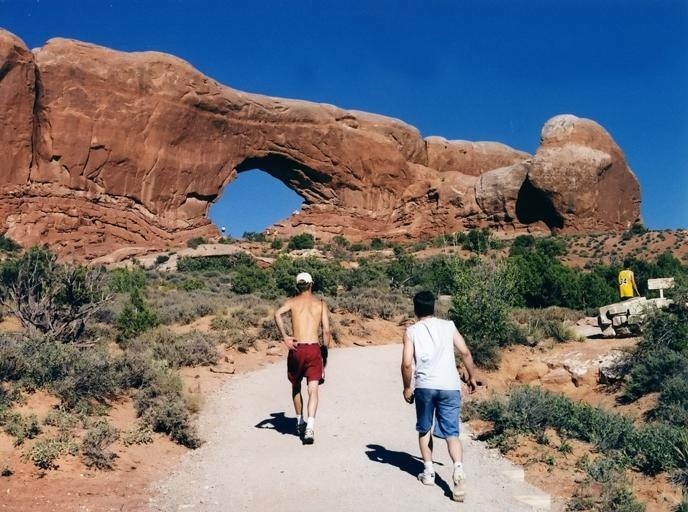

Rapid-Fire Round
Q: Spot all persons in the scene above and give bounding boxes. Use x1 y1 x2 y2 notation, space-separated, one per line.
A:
399 288 478 504
617 259 640 301
272 270 331 445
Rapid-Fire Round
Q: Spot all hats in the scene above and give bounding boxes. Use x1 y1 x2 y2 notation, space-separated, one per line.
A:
296 273 314 284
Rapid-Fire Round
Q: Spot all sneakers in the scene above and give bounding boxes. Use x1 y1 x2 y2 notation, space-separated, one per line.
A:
453 470 465 502
418 472 435 485
296 422 315 443
